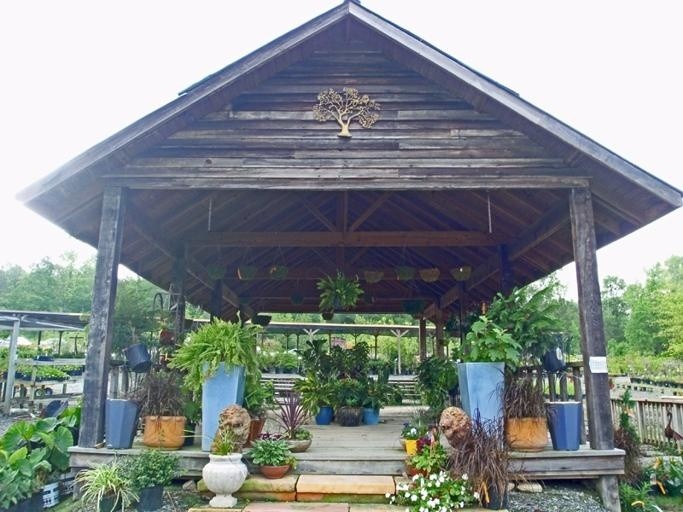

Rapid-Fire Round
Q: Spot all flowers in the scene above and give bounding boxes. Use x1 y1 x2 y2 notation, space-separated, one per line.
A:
402 418 424 438
385 471 480 512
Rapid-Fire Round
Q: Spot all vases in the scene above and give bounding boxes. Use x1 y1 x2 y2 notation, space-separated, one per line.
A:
404 439 418 453
439 339 451 347
547 402 582 451
369 266 475 316
209 262 307 326
538 333 568 373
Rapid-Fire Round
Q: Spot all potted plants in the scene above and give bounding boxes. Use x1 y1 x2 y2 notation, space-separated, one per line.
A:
102 390 140 451
202 423 248 508
88 459 142 511
273 394 311 450
82 279 156 373
456 316 548 452
140 370 190 449
171 323 269 452
315 273 367 321
454 410 509 509
291 374 403 426
254 348 308 376
251 437 298 479
1 340 87 511
137 452 184 511
404 445 438 478
